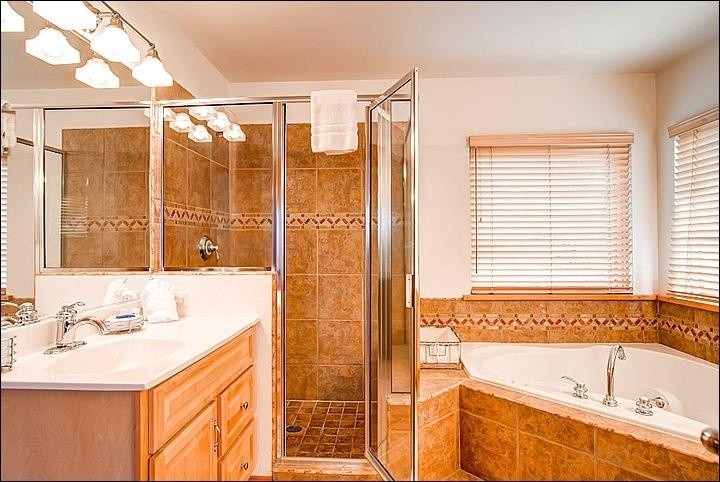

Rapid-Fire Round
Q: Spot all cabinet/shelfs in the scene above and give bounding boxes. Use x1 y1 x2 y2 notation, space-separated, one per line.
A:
145 329 257 481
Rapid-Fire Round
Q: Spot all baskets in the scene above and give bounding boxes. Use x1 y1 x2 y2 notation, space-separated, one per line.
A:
420 324 462 364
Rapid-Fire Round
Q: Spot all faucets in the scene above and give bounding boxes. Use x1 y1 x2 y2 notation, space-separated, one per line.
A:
0 300 39 327
56 300 110 347
602 343 626 408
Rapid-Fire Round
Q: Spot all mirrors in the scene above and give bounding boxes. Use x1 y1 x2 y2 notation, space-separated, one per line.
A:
0 0 153 329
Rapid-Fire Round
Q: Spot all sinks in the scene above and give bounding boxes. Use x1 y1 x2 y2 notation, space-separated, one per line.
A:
49 336 189 375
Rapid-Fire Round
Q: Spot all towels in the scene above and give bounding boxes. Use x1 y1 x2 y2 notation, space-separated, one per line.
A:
142 277 179 323
310 89 358 156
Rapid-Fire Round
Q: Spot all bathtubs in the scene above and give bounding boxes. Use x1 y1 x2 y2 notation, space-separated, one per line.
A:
458 342 719 481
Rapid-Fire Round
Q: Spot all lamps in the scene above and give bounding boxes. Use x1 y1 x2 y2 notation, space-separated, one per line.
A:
163 107 212 143
0 0 120 90
31 0 174 89
186 106 246 143
